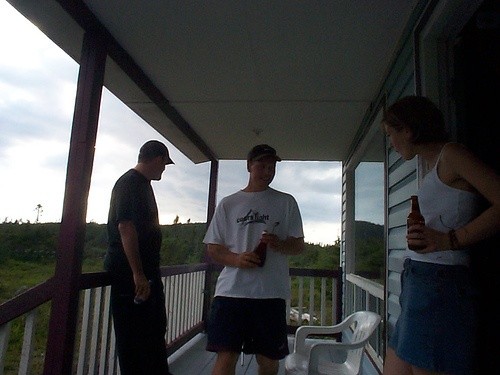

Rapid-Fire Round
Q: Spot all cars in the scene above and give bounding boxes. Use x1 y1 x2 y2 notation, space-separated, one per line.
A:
289 307 318 326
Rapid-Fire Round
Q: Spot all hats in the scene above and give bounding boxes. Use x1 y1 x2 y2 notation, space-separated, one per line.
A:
140 141 175 165
247 143 281 162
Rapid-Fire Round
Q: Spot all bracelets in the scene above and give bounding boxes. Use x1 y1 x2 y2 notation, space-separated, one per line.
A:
439 215 469 252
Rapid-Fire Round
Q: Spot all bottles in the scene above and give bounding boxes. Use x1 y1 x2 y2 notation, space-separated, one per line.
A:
247 229 270 268
133 279 152 304
407 194 426 251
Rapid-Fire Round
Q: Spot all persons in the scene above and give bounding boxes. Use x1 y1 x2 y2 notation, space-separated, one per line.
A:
107 139 176 375
201 145 306 375
379 95 499 375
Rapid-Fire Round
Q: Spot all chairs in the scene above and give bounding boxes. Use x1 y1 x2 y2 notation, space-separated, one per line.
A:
282 310 382 375
